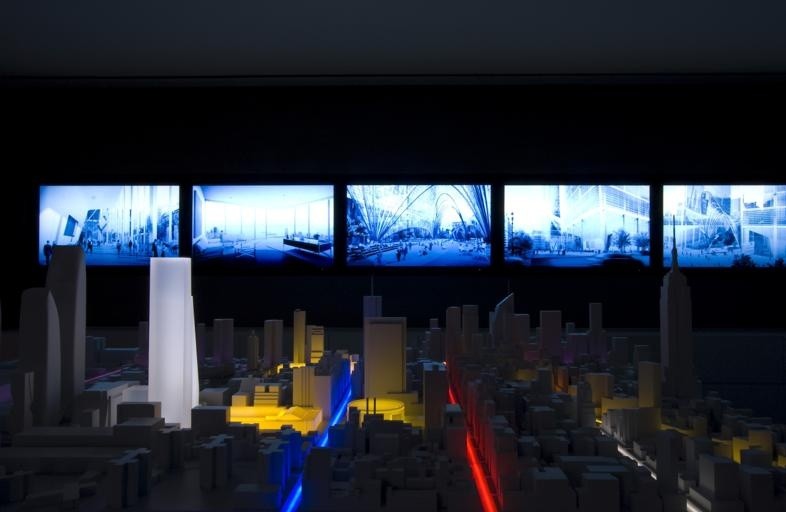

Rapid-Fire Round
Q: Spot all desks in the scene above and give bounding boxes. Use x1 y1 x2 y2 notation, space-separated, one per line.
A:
284 235 332 257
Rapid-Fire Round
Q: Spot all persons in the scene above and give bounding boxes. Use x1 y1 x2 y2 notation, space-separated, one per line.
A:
42 237 167 264
387 238 486 262
